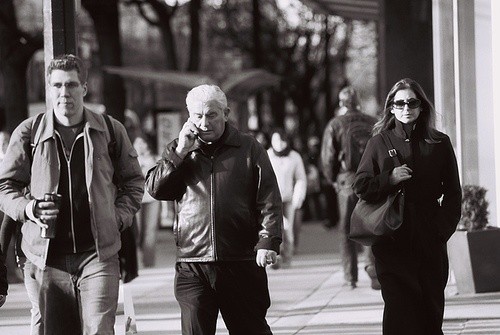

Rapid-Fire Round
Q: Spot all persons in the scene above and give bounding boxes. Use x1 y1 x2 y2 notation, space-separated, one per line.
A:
1 131 15 308
262 129 308 268
350 77 462 335
147 84 286 335
321 85 383 290
133 132 159 270
0 53 144 335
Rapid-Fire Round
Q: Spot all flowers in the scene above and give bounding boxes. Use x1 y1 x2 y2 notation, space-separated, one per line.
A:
459 185 488 231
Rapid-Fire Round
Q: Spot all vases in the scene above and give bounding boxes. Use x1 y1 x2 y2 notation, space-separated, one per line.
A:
447 225 500 293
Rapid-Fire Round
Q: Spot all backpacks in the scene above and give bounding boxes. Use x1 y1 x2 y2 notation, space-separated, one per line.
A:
338 114 373 172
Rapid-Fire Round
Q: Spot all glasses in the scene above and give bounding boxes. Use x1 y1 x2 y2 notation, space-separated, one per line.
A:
49 81 79 88
389 98 421 108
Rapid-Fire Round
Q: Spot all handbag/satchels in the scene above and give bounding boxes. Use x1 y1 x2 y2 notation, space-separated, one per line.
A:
348 132 405 246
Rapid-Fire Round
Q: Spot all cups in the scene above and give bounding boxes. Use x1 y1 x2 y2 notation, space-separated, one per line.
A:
39 193 63 239
